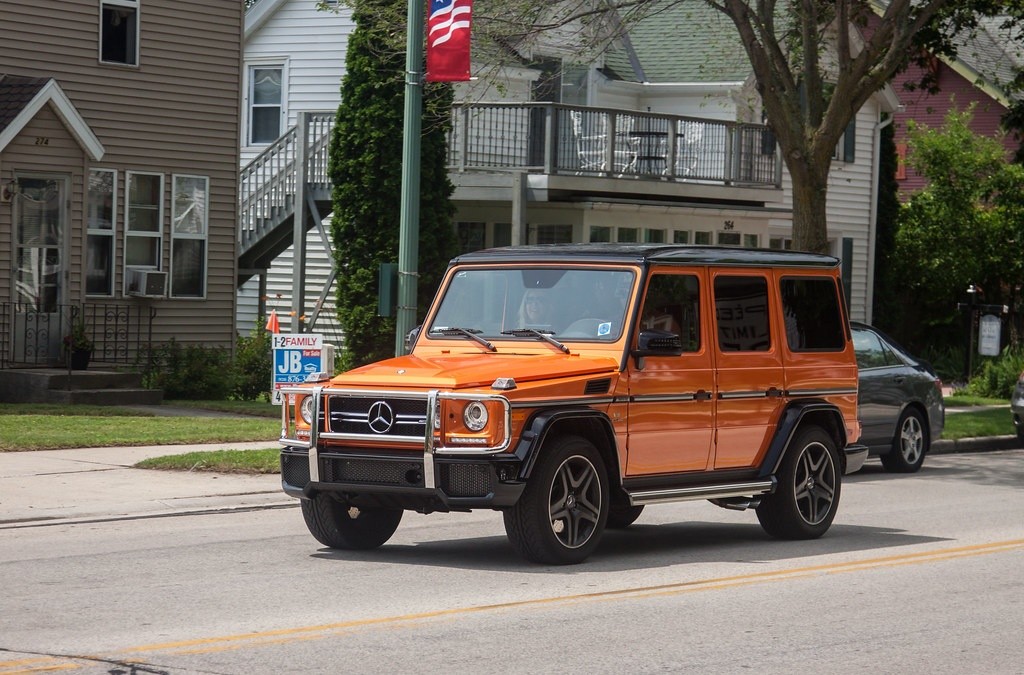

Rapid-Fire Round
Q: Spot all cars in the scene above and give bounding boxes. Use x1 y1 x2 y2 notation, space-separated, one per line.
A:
849 321 946 473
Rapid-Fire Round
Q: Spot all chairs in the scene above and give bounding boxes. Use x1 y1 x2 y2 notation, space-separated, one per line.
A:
570 111 705 183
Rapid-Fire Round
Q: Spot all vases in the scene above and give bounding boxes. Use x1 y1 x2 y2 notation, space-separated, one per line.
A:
69 349 91 370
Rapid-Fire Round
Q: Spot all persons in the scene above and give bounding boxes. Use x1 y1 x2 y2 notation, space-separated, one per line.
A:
518 287 566 336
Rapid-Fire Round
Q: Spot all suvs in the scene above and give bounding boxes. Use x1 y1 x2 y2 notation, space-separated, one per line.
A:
279 242 861 566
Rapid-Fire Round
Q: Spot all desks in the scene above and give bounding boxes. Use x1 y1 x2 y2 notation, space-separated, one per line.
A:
617 130 685 177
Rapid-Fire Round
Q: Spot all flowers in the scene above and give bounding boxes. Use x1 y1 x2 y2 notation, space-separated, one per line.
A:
62 307 95 350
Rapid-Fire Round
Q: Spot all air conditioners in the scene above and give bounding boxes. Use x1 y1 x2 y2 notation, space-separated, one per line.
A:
126 269 169 299
